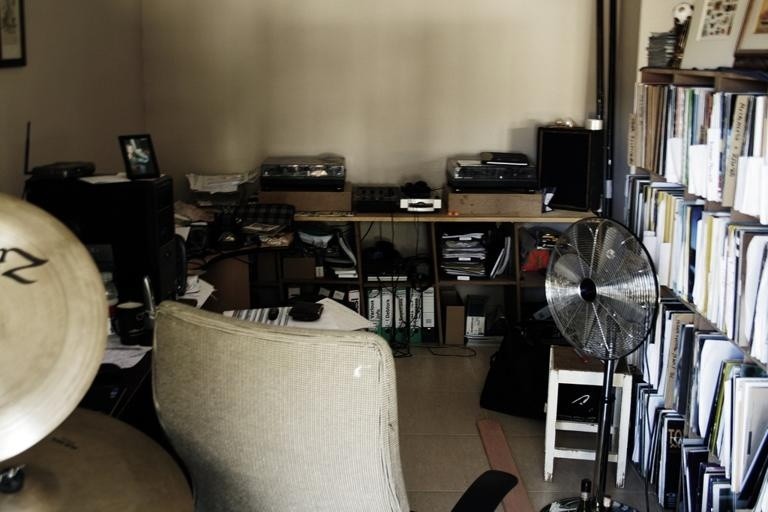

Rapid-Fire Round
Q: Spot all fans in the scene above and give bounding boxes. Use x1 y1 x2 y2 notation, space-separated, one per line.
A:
543 213 658 512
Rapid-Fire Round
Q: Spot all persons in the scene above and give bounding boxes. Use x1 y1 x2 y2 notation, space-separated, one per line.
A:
129 139 150 174
125 143 137 171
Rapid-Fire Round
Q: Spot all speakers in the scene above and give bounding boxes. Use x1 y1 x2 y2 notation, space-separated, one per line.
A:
537 127 601 212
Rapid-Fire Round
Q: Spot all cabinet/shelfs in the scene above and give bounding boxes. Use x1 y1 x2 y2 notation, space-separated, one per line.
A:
626 67 765 511
199 208 602 348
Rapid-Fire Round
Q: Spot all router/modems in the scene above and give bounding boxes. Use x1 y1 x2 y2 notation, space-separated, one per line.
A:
22 121 96 177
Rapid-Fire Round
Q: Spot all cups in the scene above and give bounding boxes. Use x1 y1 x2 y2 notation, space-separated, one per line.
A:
115 300 146 347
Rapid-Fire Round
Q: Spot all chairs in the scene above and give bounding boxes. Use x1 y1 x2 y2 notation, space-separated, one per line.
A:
152 299 518 511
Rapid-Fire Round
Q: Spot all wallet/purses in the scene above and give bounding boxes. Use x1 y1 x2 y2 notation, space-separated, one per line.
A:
288 303 323 322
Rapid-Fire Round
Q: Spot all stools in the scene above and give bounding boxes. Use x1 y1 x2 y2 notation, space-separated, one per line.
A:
543 345 632 489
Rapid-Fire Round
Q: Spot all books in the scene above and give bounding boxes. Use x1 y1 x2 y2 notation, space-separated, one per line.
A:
620 79 768 511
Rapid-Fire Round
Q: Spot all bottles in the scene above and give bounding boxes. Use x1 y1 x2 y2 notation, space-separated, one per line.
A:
101 271 120 338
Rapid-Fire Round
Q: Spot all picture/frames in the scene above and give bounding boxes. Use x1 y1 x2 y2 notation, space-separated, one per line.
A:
679 0 768 70
117 132 162 180
0 1 27 68
